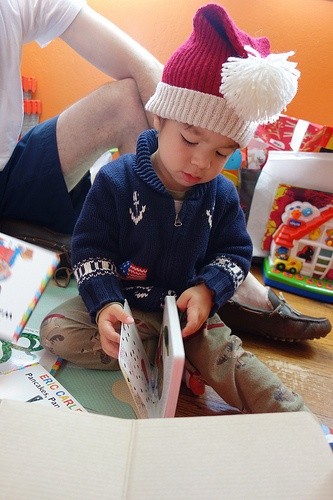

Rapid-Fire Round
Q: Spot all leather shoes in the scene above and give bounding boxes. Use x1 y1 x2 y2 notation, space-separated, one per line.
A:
218 286 332 342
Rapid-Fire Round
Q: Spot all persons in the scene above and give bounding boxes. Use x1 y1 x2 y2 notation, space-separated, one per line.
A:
0 1 332 343
40 4 333 462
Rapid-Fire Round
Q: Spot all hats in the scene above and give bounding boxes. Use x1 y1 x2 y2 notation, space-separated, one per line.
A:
144 4 301 148
